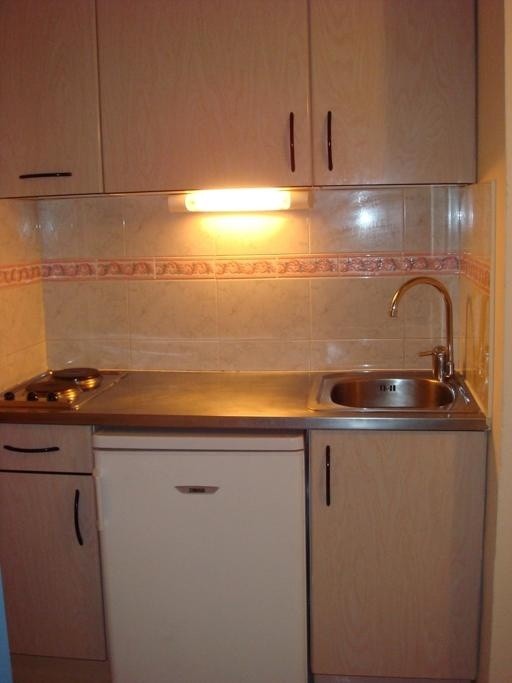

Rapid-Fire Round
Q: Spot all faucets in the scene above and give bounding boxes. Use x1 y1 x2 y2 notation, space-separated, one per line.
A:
386 273 454 383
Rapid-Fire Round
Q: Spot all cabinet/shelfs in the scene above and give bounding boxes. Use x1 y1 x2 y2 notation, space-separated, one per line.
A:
1 0 104 198
0 420 108 681
306 426 487 682
94 4 480 195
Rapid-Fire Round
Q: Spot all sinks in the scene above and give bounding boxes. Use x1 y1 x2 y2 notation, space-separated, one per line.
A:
325 373 460 415
